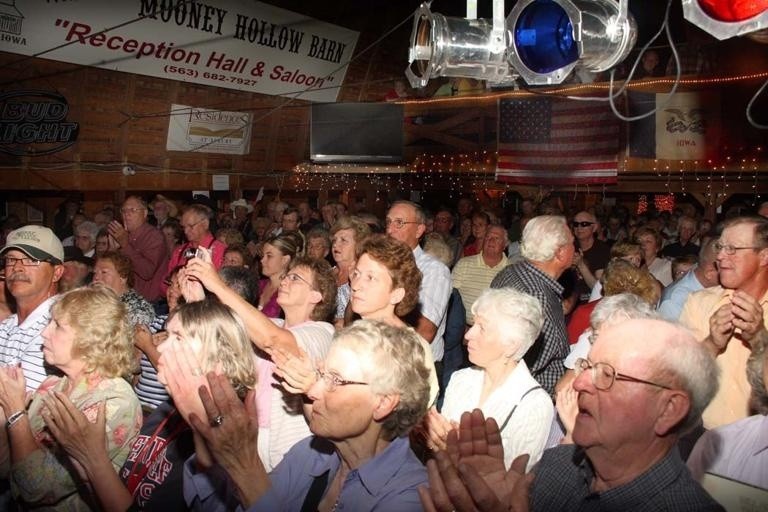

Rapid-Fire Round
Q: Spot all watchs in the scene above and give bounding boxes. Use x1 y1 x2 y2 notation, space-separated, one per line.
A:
3 410 25 428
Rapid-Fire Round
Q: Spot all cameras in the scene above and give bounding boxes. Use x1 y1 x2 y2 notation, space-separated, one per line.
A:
184 247 213 260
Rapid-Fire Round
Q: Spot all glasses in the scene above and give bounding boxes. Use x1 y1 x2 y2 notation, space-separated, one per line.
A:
385 219 423 228
182 218 205 231
278 273 320 295
121 207 147 215
714 243 762 255
314 368 374 391
4 257 54 268
574 359 676 394
573 221 595 227
675 270 687 275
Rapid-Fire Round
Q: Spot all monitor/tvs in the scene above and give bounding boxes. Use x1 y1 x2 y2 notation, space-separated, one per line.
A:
310 101 405 163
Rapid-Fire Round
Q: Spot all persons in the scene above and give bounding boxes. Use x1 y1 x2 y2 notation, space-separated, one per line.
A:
460 211 491 258
268 208 306 254
92 226 121 259
510 199 538 241
40 301 262 511
633 227 672 288
559 210 611 303
123 264 195 418
698 219 713 235
661 217 700 262
229 198 256 225
422 210 435 235
451 223 514 346
297 202 320 234
673 217 768 398
220 244 254 272
304 230 331 260
459 218 475 246
54 244 96 294
73 220 99 259
628 209 660 227
271 236 440 457
555 295 656 393
106 195 168 303
62 213 97 247
148 194 183 235
204 265 260 316
1 224 79 412
2 282 146 509
600 211 627 240
216 214 246 245
310 209 324 223
326 218 376 327
86 250 158 330
435 210 461 264
243 216 273 256
417 320 723 512
488 214 577 399
672 207 683 217
608 241 644 268
313 202 338 230
383 199 452 373
437 284 555 477
564 202 629 214
599 258 661 313
332 201 348 220
180 244 336 476
657 236 720 322
188 318 435 512
151 204 227 316
669 255 693 282
52 195 87 241
659 210 671 221
253 238 305 318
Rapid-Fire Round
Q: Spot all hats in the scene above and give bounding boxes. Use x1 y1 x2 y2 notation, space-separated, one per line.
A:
499 191 523 215
62 190 83 207
190 195 218 211
230 199 252 213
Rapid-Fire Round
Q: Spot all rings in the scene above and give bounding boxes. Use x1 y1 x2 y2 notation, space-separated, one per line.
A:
209 416 223 428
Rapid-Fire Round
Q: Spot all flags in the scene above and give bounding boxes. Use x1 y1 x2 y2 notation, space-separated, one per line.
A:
495 93 625 186
626 89 724 162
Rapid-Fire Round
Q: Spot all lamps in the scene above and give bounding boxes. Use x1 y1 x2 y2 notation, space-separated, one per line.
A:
291 150 494 202
674 0 767 49
403 1 521 91
506 1 640 92
651 143 767 207
